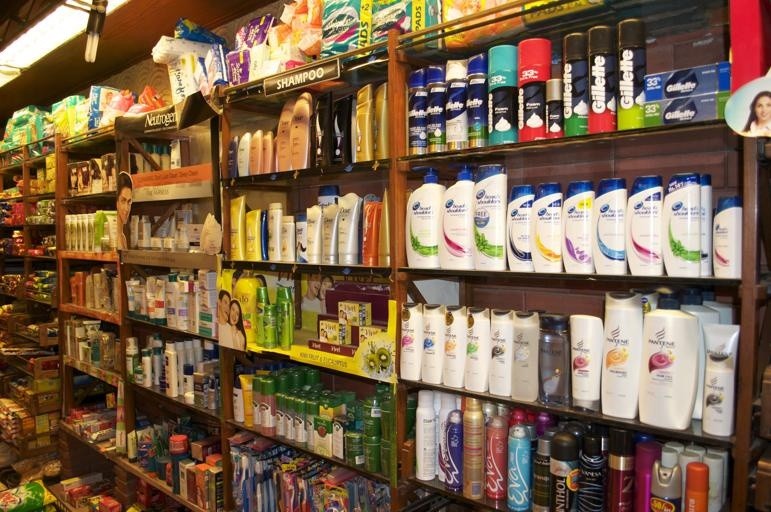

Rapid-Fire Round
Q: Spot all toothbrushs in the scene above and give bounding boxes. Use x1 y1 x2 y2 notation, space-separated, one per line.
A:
228 429 391 511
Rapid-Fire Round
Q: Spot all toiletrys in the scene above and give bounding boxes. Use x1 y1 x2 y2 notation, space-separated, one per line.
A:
399 32 742 512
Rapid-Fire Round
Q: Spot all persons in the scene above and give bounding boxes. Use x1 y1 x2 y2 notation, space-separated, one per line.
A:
740 90 771 136
218 270 348 351
115 171 133 251
68 154 116 194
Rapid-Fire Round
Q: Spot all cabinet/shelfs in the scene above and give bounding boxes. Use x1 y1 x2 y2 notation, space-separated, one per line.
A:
0 0 770 512
